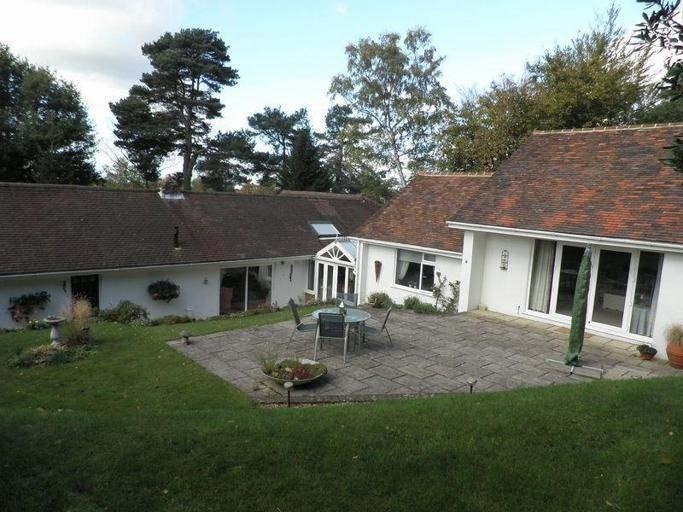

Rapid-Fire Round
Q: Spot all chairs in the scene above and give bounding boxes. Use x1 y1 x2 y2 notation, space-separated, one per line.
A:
286 293 395 368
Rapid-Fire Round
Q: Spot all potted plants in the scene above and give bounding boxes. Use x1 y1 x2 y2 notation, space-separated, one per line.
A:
665 322 683 370
637 344 657 361
